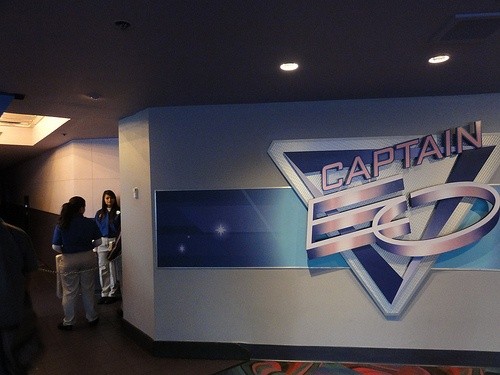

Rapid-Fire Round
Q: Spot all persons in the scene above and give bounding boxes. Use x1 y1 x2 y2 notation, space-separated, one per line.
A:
95 190 122 297
0 217 39 375
52 196 103 330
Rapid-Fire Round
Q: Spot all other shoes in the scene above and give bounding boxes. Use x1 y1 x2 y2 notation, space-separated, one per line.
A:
88 319 99 325
57 322 72 330
98 297 118 304
